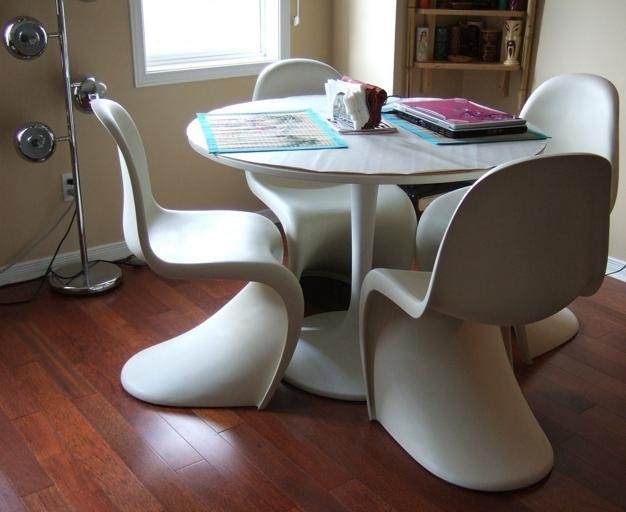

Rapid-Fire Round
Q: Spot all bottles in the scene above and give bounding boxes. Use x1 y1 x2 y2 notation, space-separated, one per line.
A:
435 17 523 67
416 16 430 62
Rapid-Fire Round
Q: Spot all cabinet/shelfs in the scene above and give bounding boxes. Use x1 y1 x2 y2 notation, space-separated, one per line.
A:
406 1 537 118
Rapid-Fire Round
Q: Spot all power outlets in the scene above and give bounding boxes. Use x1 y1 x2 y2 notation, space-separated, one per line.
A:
61 172 75 201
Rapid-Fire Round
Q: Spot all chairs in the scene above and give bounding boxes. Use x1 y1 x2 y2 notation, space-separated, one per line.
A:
87 96 308 411
356 151 614 492
244 57 418 282
415 73 621 360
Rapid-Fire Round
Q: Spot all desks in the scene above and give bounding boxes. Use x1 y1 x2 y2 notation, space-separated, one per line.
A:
185 94 550 404
